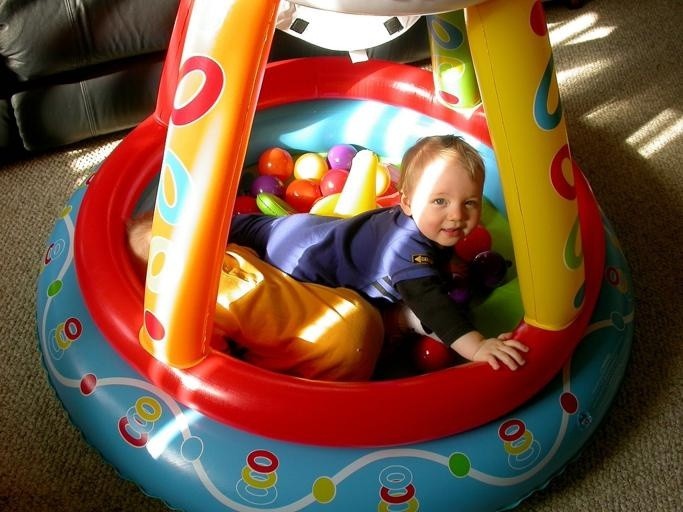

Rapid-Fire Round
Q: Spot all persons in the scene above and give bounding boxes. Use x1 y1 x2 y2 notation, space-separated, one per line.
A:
228 135 530 371
127 216 386 383
